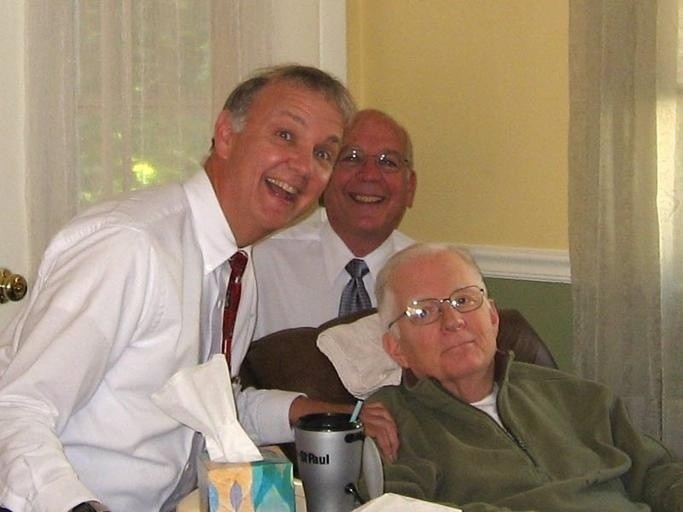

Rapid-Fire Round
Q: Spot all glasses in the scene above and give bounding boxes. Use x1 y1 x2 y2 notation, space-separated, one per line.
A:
338 143 412 175
389 285 488 330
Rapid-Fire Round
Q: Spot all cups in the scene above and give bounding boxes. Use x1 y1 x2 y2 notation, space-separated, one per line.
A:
294 399 365 512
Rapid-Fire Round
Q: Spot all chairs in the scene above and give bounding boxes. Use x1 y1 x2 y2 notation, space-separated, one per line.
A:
236 310 556 469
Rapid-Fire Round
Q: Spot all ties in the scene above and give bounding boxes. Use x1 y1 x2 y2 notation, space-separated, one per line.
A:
339 259 372 318
222 249 252 376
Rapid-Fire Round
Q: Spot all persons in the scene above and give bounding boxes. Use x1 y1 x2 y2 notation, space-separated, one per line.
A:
0 66 401 512
359 243 683 512
251 111 416 339
